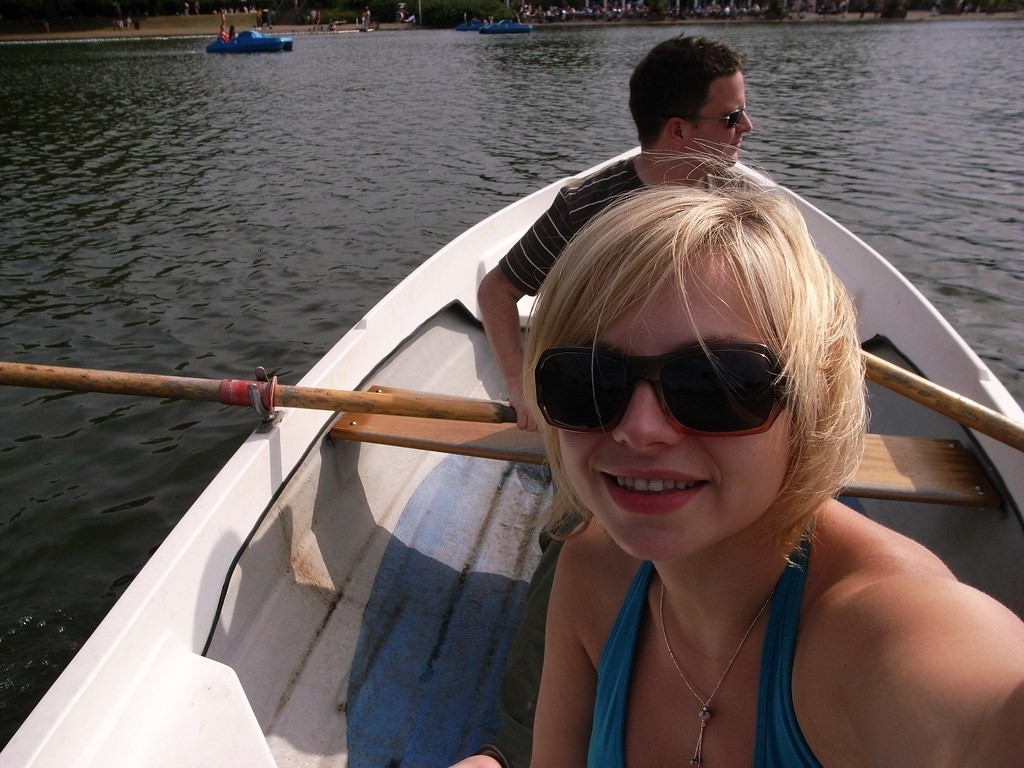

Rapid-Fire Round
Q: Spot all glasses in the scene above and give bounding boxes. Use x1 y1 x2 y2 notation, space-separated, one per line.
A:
534 341 797 438
663 103 746 130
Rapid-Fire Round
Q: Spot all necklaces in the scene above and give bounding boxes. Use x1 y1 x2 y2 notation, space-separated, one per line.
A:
658 580 775 768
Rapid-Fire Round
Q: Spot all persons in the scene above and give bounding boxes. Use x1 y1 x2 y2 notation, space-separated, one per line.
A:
111 1 416 35
451 180 1024 768
226 25 238 42
511 1 765 20
218 24 228 42
477 33 868 768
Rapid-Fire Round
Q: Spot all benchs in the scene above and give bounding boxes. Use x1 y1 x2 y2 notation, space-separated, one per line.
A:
331 384 1001 510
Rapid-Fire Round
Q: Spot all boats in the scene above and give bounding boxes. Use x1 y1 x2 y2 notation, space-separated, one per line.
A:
479 19 533 34
456 21 483 31
206 31 293 54
